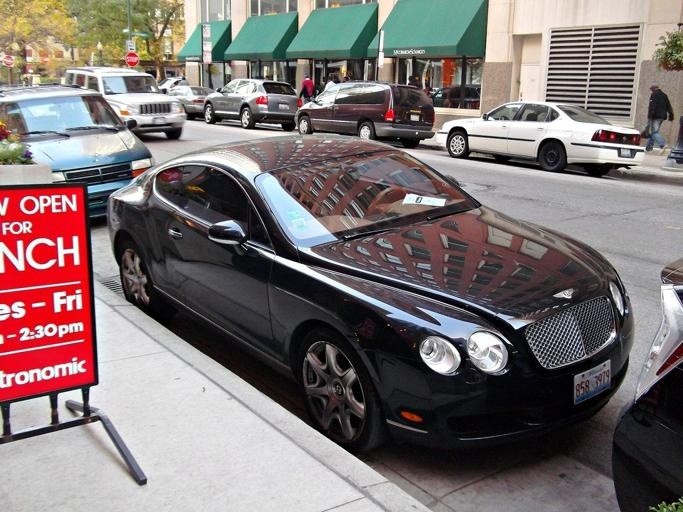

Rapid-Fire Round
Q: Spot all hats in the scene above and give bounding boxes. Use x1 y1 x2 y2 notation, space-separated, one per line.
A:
304 72 309 77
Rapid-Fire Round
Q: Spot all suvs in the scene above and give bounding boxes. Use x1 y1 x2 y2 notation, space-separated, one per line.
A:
432 84 480 110
65 66 186 139
203 79 303 131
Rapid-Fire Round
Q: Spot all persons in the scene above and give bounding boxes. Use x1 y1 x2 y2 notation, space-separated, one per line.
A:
407 75 419 88
342 76 348 84
298 72 316 105
265 76 269 80
642 84 675 156
323 76 335 91
422 85 433 96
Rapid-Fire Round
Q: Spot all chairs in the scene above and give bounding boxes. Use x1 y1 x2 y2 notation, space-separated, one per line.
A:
525 111 554 121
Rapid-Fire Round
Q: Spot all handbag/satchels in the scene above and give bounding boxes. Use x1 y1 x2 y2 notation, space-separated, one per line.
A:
641 124 651 138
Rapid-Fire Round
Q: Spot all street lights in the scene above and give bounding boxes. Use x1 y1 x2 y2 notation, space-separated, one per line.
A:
95 41 103 64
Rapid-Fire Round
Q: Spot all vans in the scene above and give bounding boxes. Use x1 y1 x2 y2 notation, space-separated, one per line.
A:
1 84 153 220
295 80 436 148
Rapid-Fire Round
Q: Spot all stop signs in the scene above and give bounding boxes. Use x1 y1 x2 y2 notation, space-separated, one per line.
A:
126 53 139 66
3 56 14 66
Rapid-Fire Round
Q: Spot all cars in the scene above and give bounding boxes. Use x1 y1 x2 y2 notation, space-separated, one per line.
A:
437 101 644 176
157 77 185 91
168 86 216 120
107 135 635 456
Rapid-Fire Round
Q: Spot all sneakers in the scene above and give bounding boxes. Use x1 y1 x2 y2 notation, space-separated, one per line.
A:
644 145 668 155
